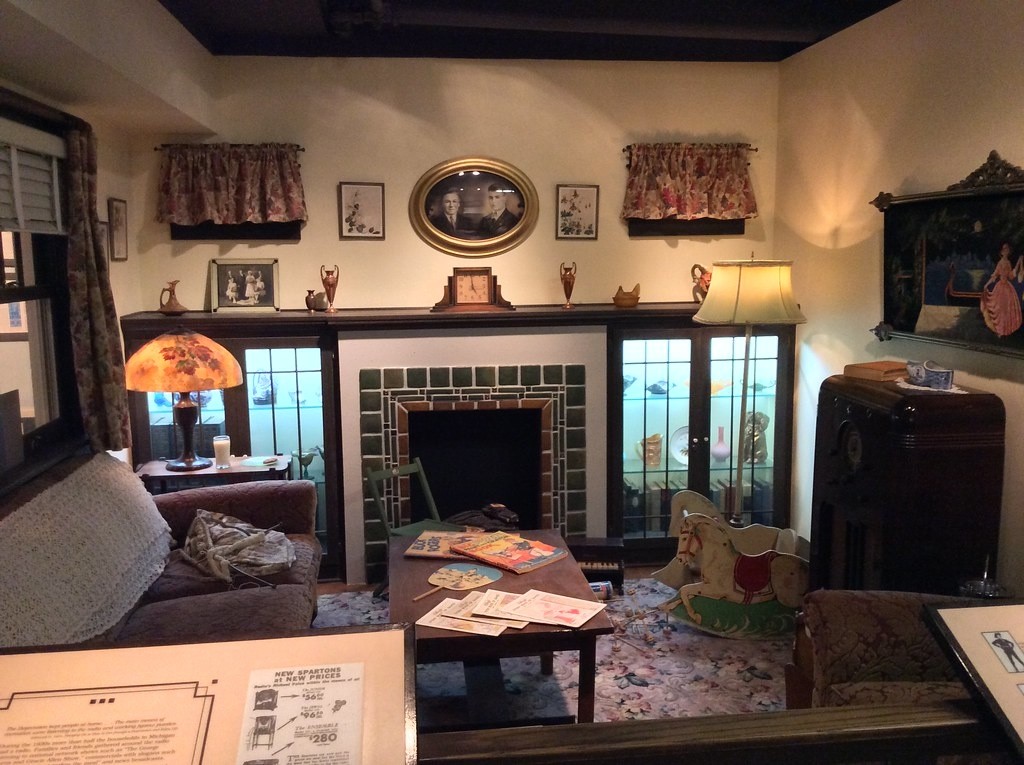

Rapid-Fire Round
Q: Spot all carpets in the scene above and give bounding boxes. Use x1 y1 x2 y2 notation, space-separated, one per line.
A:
310 576 800 724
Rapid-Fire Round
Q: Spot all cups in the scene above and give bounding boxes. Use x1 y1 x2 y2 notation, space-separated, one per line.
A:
212 435 232 470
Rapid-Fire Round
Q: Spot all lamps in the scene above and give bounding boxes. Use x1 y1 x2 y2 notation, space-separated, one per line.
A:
691 252 807 528
123 324 243 471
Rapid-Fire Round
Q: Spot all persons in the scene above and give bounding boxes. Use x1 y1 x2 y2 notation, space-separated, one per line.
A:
430 188 472 238
476 184 521 237
224 270 266 305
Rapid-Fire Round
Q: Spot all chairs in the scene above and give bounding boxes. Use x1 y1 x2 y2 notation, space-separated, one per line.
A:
366 457 467 597
783 588 1024 710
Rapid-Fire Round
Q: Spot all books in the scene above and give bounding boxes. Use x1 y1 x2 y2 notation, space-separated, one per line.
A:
451 530 568 575
402 529 520 561
844 361 909 382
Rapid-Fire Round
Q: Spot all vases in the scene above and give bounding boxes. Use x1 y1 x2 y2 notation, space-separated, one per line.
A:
711 426 730 463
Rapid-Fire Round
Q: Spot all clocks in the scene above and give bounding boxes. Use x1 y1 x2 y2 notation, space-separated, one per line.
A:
430 267 516 312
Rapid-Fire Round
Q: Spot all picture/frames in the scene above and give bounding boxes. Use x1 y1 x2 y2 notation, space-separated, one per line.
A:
869 150 1024 360
0 259 29 342
108 197 128 261
210 258 281 314
338 181 386 240
98 220 109 279
555 184 599 240
408 157 540 258
919 597 1024 765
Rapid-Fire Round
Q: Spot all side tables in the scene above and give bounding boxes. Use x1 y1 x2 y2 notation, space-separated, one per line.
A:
135 453 294 495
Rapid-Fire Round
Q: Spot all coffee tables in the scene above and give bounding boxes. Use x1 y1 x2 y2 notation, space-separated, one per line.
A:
389 528 616 731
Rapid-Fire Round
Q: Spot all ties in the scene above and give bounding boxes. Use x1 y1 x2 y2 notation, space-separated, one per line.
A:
449 216 456 232
492 217 496 223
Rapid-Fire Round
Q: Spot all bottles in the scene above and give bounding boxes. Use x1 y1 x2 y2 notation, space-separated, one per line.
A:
306 289 316 313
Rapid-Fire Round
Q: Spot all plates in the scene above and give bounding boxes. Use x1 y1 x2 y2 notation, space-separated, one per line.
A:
241 456 279 467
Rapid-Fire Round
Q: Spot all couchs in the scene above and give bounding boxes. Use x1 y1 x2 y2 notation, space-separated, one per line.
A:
0 448 322 645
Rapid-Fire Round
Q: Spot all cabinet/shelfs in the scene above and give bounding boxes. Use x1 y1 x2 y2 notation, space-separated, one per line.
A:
123 335 345 583
606 322 796 568
809 376 1006 593
